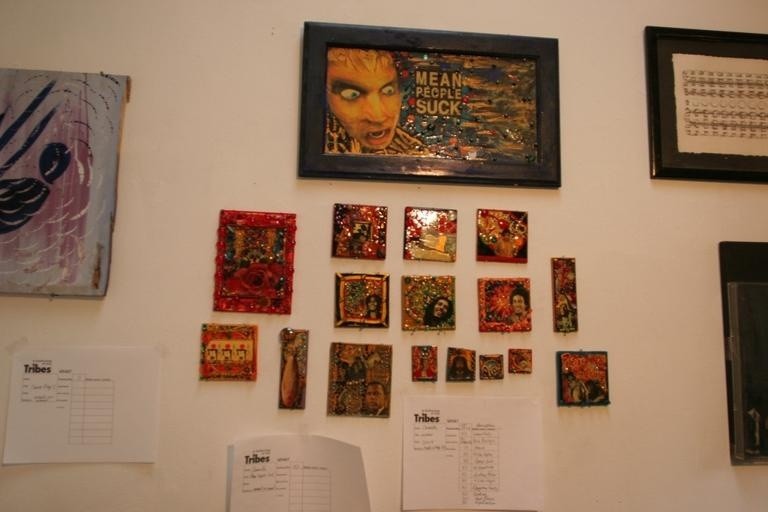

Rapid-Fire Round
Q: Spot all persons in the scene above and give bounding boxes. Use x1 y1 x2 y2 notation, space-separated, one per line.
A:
364 381 388 415
365 294 381 321
509 286 528 323
425 296 452 325
327 45 431 156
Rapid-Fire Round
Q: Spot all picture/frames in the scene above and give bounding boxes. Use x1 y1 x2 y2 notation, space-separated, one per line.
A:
556 352 610 406
299 22 563 186
644 25 768 184
335 273 390 328
